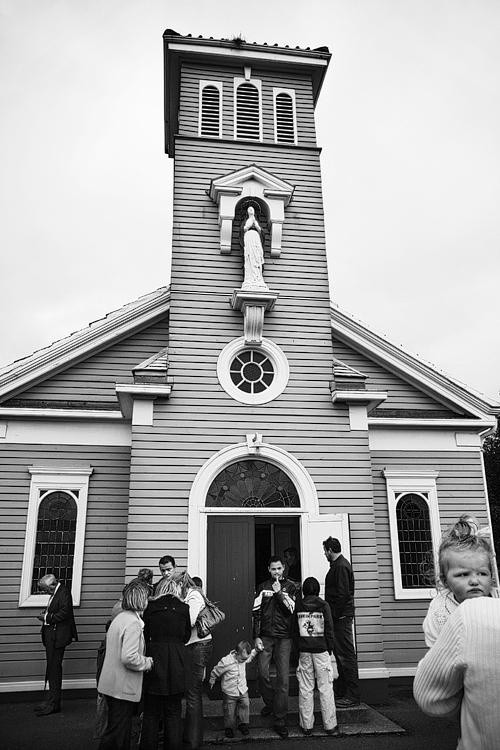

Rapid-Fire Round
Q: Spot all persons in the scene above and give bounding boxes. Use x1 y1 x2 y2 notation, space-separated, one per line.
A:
209 640 263 736
32 574 78 716
284 547 302 583
253 555 298 737
322 537 361 708
97 555 212 750
239 205 269 291
413 512 500 750
292 577 340 735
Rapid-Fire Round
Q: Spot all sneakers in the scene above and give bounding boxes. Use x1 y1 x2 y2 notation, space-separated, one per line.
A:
335 696 361 708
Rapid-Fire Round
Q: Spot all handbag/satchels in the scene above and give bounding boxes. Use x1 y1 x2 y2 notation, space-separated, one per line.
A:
195 603 226 639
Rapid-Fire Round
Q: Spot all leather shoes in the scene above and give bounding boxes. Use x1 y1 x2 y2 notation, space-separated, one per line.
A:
34 702 63 716
261 704 274 716
237 724 250 736
274 725 289 738
302 727 311 735
325 728 336 734
225 727 235 738
